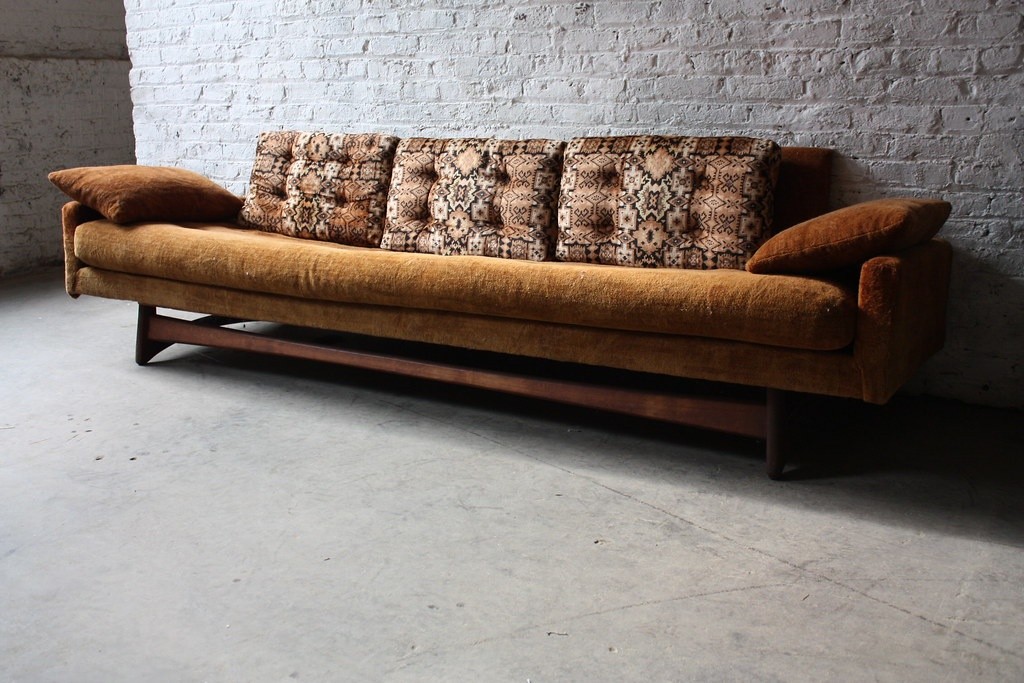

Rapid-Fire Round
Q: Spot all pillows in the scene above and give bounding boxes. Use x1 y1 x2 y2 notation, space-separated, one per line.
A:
746 196 954 274
47 164 248 229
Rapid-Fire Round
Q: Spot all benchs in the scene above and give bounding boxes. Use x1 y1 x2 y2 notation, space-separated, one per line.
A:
61 130 953 481
